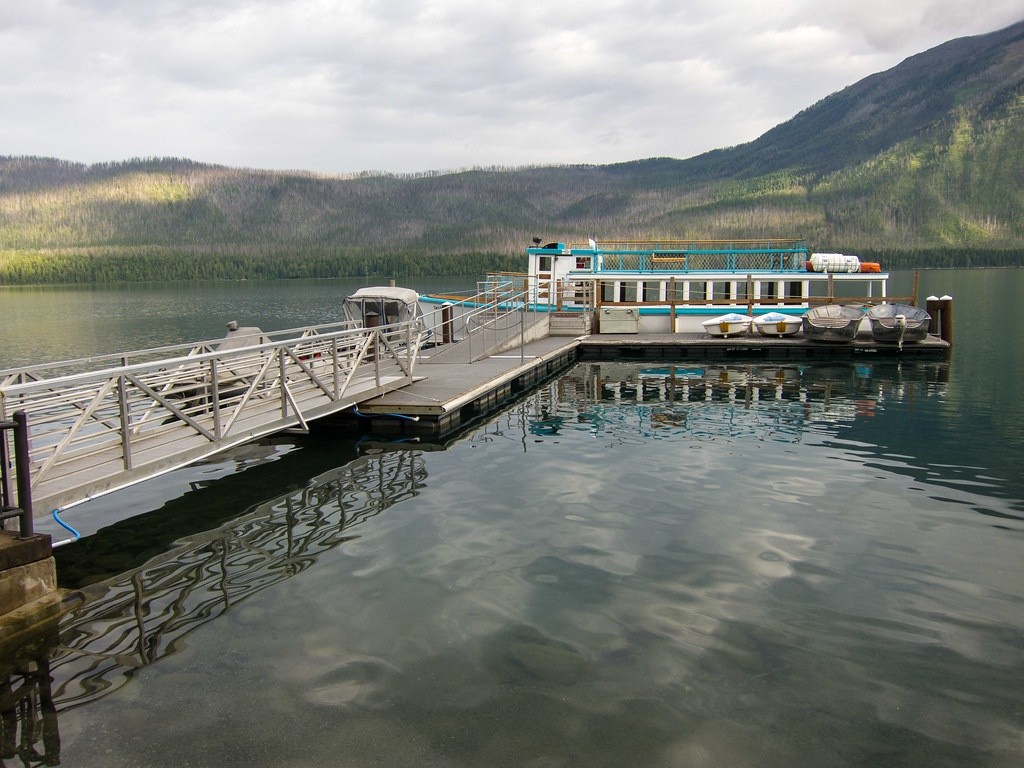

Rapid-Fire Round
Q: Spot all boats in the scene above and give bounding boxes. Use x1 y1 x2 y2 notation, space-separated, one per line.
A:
800 302 867 342
701 312 752 339
753 312 803 339
866 300 933 343
413 230 916 349
181 281 433 400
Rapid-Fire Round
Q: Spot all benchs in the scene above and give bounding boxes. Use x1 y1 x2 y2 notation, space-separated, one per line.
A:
876 316 920 327
812 317 850 326
